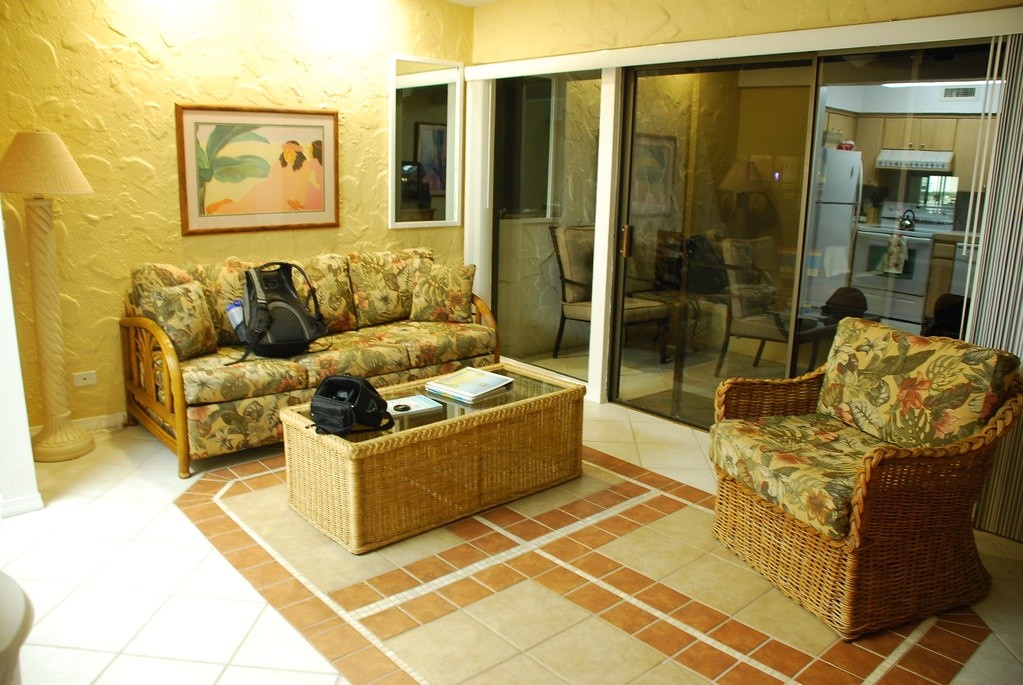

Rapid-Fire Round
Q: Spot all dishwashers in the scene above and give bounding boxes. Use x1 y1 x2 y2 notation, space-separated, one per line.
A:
950 242 979 298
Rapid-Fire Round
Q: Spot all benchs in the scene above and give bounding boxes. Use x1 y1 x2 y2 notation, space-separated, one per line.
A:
119 286 500 478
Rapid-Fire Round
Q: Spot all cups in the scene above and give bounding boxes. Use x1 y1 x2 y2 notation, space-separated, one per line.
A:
225 300 244 329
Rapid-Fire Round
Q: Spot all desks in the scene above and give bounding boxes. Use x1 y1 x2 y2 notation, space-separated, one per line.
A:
713 304 882 382
278 361 588 554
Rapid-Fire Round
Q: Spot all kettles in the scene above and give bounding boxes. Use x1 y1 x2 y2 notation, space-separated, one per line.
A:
897 209 915 231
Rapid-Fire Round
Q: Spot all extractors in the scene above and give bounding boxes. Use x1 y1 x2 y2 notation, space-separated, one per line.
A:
875 149 954 172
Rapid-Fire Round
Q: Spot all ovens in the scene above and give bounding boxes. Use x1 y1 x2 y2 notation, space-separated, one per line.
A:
849 231 935 334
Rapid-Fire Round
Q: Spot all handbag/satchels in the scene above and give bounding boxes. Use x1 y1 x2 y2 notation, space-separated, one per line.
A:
822 287 866 319
309 375 395 435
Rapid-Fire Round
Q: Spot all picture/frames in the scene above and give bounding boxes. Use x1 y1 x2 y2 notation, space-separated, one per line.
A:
414 120 448 195
629 132 679 217
175 100 339 237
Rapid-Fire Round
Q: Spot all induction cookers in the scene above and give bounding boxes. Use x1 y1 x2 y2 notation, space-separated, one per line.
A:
857 200 956 239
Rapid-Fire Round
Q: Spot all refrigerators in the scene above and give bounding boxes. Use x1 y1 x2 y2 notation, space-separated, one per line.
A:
798 147 863 309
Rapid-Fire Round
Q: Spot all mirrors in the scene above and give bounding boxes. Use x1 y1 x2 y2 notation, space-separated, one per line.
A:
387 50 466 229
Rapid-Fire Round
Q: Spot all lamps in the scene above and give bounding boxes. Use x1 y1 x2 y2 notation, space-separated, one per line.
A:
0 130 97 463
720 161 768 240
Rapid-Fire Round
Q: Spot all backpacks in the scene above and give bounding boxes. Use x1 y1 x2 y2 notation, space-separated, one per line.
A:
226 262 332 364
684 235 727 291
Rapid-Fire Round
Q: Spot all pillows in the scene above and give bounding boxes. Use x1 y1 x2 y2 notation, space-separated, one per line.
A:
408 257 477 322
132 247 435 342
153 281 217 362
816 316 1020 451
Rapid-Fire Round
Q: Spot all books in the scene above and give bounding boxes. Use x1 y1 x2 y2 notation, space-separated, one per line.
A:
386 394 443 417
426 365 515 405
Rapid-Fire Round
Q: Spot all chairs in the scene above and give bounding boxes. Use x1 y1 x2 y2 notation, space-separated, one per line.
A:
708 317 1022 643
652 228 775 377
712 236 835 379
547 225 671 362
